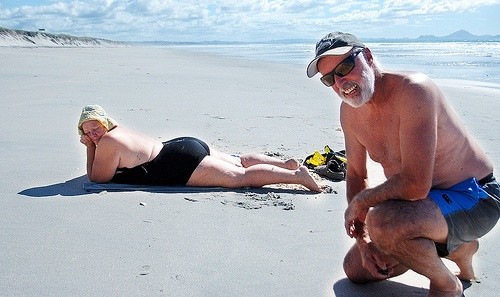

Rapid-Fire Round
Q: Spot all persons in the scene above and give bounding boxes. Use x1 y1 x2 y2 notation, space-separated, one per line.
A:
78 105 326 193
306 31 500 297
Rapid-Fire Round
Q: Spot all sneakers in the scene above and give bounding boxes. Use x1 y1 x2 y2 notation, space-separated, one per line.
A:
340 162 347 173
313 161 344 181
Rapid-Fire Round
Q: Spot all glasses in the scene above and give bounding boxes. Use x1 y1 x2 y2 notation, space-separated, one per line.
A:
320 48 363 87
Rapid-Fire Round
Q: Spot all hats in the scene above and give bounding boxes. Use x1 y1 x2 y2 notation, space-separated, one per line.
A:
306 31 365 78
